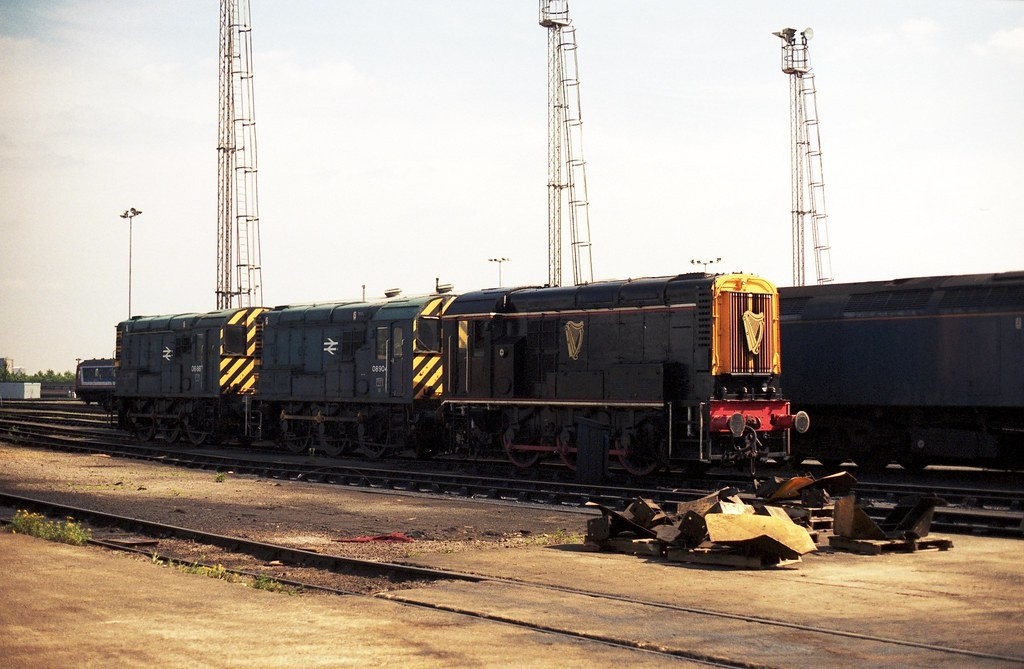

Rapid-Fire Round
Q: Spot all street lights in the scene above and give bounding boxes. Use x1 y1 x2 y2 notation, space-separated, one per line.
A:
691 257 722 274
119 207 142 319
489 256 510 289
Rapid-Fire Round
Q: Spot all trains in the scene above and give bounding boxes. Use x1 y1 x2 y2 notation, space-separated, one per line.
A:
107 273 809 485
76 360 117 407
778 270 1023 474
